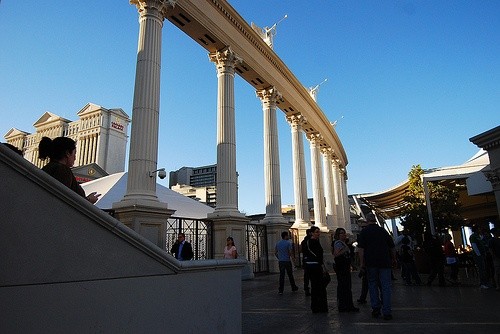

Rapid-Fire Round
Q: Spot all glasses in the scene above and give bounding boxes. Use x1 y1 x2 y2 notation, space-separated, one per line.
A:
314 230 320 233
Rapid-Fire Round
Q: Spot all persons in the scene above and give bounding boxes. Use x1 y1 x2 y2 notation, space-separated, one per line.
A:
301 213 500 320
223 237 238 259
274 232 298 295
171 233 193 261
0 137 101 204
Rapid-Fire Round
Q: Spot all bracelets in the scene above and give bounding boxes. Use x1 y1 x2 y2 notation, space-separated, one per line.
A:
87 196 90 201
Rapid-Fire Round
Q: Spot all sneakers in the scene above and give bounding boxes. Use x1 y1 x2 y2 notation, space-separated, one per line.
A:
449 279 458 285
480 284 489 288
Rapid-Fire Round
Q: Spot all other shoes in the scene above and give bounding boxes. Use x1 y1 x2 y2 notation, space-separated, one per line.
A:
291 287 298 291
279 291 283 295
371 310 392 321
339 307 360 312
357 299 366 303
